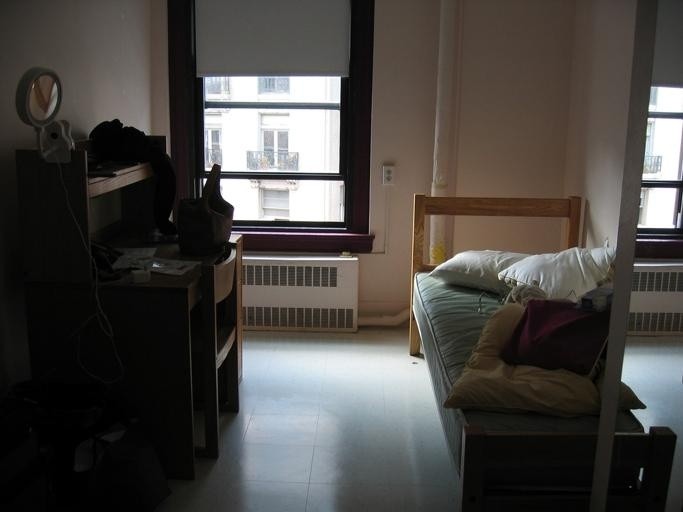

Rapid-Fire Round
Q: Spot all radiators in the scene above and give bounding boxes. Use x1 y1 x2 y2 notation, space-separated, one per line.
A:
242 257 359 332
627 263 683 336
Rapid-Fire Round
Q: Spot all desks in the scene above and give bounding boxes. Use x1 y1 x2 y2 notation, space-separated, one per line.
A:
16 136 243 481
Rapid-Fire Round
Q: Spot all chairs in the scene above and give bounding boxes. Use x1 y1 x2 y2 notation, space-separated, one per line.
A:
204 242 242 450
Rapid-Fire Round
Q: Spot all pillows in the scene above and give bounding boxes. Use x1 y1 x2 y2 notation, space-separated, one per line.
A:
429 240 617 417
618 381 646 409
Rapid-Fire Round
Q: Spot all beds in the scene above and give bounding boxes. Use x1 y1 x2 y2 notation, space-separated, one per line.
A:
606 410 677 512
408 193 602 512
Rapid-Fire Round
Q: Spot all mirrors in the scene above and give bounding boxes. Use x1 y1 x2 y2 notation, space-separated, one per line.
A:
15 68 62 153
589 0 683 512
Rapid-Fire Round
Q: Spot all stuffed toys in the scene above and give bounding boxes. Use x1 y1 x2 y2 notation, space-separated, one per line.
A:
505 281 547 307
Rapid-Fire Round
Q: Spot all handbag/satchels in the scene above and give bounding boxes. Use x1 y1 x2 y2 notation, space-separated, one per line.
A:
177 162 234 259
500 298 606 378
